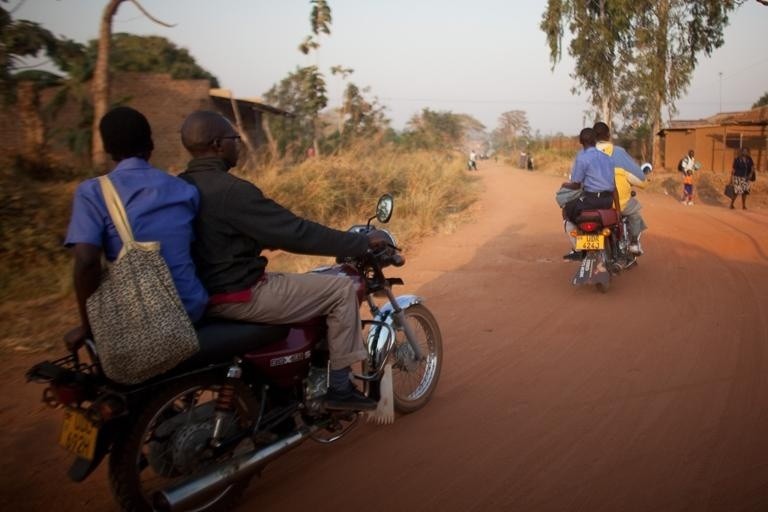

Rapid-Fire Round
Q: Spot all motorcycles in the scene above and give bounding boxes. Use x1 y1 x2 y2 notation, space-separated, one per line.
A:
30 184 440 510
554 163 652 293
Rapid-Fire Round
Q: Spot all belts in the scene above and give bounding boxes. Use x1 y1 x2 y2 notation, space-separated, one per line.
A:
584 191 612 198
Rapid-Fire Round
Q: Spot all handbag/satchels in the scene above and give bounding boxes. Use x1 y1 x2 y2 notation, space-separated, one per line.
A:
85 239 200 384
557 183 583 208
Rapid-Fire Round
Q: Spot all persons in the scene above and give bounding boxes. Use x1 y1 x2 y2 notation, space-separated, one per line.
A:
681 169 694 205
526 153 533 171
593 122 646 255
559 128 615 262
172 109 388 411
64 105 210 354
468 150 477 170
682 149 696 176
729 146 755 210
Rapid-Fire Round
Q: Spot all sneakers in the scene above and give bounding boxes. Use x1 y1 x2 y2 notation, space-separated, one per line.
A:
325 379 378 410
630 241 639 254
563 249 585 258
